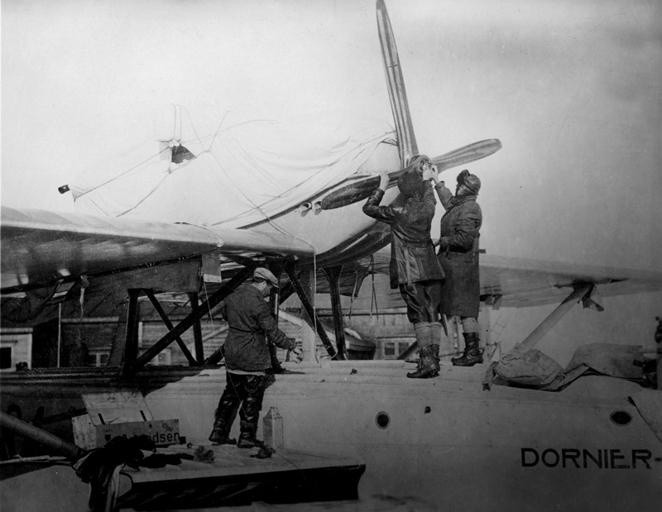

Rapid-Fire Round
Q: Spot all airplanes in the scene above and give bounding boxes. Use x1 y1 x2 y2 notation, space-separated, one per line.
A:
0 0 662 465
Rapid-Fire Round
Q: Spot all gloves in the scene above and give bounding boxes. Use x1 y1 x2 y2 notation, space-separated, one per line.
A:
423 163 440 180
379 171 390 192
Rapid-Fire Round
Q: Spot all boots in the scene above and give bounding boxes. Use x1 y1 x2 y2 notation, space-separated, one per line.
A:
407 344 440 378
451 332 484 366
209 415 264 448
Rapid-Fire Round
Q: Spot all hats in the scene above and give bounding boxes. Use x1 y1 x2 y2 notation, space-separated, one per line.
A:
457 170 481 194
397 171 424 196
254 267 279 288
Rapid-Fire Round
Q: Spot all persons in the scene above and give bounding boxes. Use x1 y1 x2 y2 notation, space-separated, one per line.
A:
209 267 302 448
431 165 483 366
362 167 446 378
